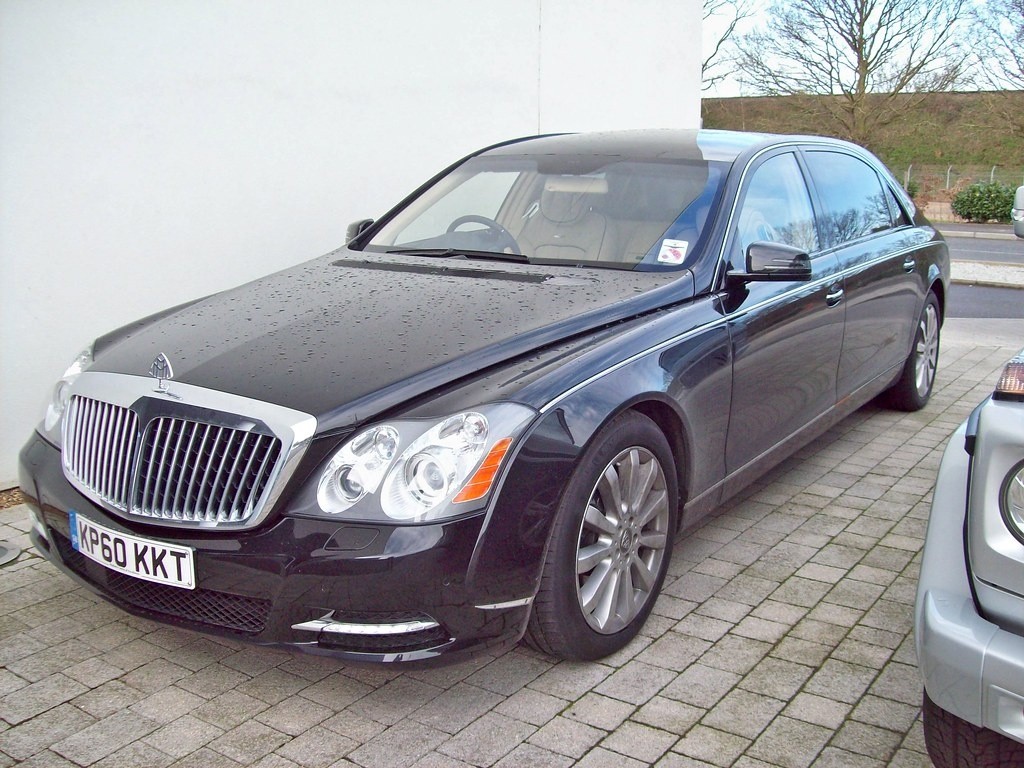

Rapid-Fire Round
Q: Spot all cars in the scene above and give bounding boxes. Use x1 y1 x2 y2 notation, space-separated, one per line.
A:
912 352 1024 765
18 130 951 669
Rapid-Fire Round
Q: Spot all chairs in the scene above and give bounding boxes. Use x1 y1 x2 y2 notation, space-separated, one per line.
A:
503 190 786 268
